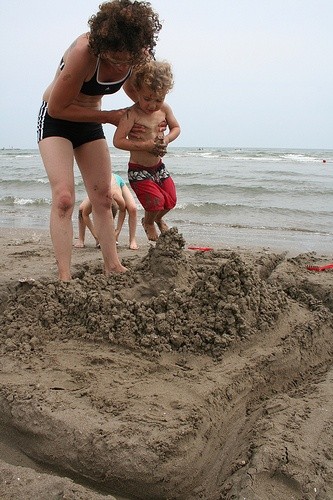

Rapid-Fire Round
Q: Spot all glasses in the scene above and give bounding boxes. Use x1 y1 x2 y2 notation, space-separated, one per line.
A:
104 53 148 67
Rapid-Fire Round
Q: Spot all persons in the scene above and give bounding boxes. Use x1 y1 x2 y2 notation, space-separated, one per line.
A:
113 61 180 241
74 173 140 250
37 0 162 284
